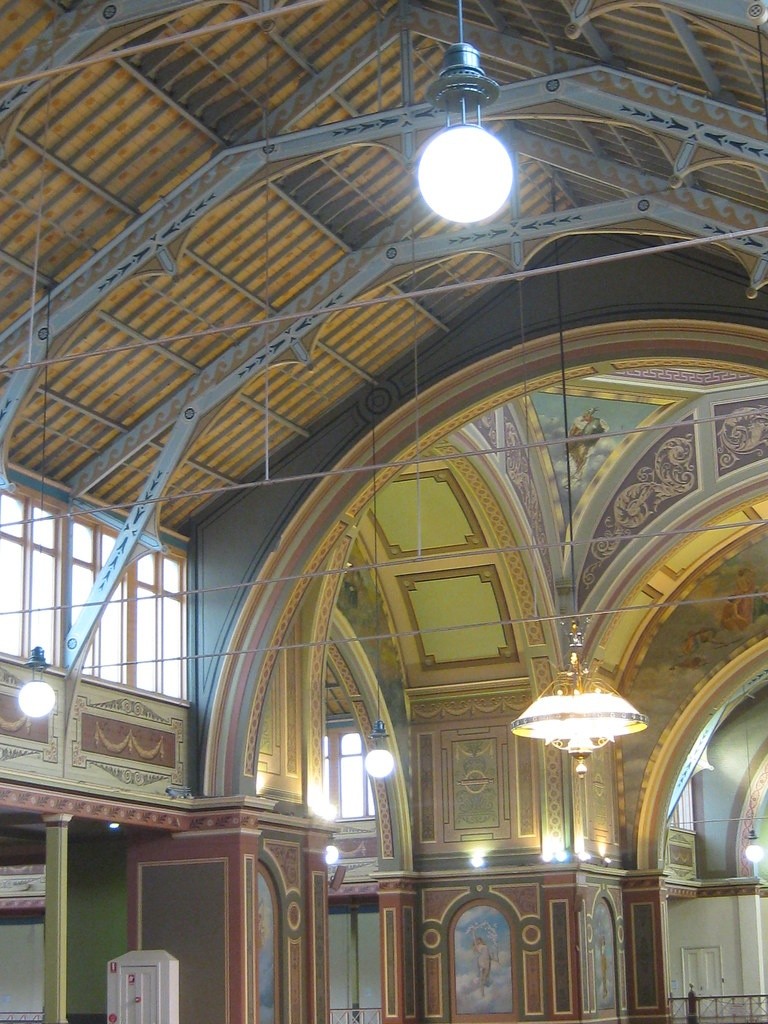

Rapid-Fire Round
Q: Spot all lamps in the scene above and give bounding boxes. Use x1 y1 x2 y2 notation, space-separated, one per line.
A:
741 683 764 863
325 686 340 866
364 376 396 780
18 287 58 720
503 165 653 782
417 0 515 227
